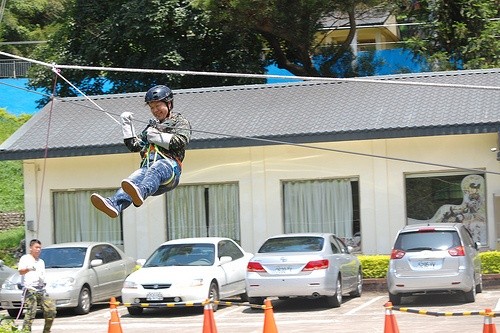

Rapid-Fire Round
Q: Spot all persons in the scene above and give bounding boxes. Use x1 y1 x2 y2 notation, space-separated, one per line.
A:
18 239 56 333
91 85 192 218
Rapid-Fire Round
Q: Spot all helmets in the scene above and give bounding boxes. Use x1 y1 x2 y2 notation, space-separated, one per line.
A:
145 85 173 102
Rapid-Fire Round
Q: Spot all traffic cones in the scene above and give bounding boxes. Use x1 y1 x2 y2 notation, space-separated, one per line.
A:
263 298 278 333
202 299 218 333
108 296 122 333
383 301 400 333
482 308 496 333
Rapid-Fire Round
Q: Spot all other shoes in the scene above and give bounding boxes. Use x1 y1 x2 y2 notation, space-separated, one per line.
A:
120 178 144 206
90 193 119 218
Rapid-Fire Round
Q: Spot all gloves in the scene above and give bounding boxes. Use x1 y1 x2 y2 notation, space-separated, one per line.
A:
145 127 173 150
120 111 137 138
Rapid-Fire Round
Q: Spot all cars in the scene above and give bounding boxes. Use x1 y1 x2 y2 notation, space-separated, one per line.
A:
245 231 364 308
386 216 483 305
0 259 18 311
121 236 254 314
1 241 143 317
